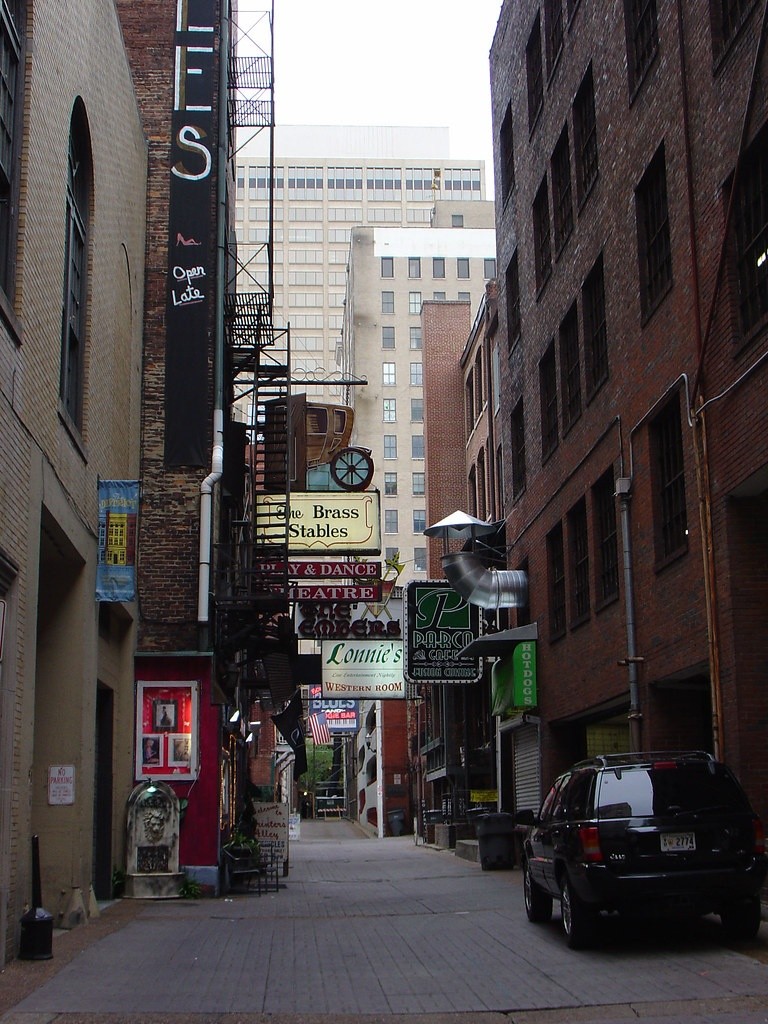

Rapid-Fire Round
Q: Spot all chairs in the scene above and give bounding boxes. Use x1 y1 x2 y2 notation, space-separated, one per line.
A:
224 841 279 897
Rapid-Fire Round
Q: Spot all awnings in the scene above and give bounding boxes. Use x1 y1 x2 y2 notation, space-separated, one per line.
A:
456 622 537 657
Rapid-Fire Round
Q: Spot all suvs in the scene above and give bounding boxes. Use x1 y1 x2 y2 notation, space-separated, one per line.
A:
513 750 768 951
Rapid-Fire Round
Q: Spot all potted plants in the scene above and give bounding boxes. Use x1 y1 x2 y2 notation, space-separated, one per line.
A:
230 827 258 869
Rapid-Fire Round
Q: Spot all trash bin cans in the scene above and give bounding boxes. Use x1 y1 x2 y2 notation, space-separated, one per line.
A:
425 809 442 823
387 809 404 836
466 808 490 840
19 836 55 960
477 813 516 871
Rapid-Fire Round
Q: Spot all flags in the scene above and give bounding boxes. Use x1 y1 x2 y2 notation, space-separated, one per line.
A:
271 688 308 784
309 712 331 746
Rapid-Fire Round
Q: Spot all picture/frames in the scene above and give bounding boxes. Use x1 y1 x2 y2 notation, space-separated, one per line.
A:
168 733 191 766
152 700 178 730
142 734 164 768
136 681 198 780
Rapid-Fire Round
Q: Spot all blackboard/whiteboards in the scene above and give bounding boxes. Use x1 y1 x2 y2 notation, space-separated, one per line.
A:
288 813 301 841
248 801 289 863
